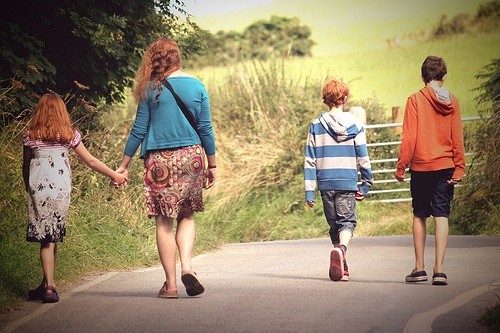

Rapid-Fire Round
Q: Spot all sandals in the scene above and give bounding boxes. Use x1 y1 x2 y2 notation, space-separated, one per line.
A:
28 283 59 303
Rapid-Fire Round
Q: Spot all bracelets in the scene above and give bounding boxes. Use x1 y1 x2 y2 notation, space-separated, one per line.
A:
207 166 217 169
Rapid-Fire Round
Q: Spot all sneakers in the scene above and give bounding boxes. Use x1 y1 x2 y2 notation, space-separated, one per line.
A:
431 272 448 285
405 269 428 282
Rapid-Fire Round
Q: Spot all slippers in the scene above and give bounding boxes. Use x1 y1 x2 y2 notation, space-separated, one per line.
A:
159 281 178 298
181 272 205 296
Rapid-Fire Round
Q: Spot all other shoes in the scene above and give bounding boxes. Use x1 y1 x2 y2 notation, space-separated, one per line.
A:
340 258 350 281
329 245 346 281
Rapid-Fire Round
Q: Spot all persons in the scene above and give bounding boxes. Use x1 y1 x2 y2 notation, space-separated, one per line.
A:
111 39 217 300
395 56 465 286
24 92 129 304
304 81 373 282
